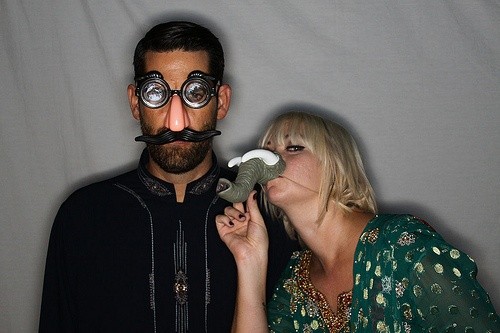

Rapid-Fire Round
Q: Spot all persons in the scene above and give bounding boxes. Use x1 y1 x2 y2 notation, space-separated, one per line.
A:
38 20 304 333
214 109 500 333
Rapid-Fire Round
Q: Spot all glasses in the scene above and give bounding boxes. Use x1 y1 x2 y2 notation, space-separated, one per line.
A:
134 77 220 107
